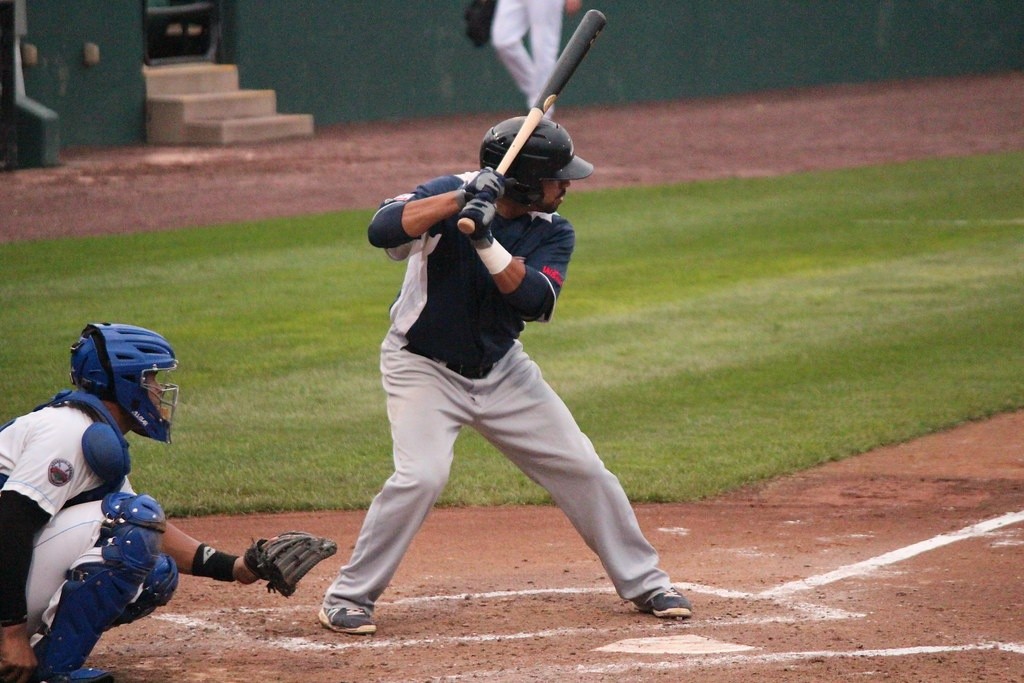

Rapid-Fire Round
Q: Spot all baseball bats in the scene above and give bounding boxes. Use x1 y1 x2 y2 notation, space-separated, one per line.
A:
457 8 607 234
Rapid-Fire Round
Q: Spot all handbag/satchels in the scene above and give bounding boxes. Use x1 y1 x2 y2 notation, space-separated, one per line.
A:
462 0 499 47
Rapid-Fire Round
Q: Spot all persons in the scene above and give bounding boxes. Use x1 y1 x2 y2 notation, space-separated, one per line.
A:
1 320 299 683
313 114 693 635
460 0 584 124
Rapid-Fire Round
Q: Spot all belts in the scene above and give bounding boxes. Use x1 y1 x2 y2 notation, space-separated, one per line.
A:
403 342 493 378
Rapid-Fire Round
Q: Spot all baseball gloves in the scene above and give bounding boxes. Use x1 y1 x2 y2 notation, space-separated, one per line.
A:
243 530 337 599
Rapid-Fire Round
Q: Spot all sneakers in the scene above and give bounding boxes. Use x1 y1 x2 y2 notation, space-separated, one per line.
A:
30 668 114 683
318 606 377 634
639 587 692 619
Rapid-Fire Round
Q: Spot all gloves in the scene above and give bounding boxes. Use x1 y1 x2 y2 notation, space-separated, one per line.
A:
458 166 505 211
458 192 493 249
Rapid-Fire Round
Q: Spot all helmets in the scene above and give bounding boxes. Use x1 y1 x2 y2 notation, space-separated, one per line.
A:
69 322 176 441
479 116 593 205
